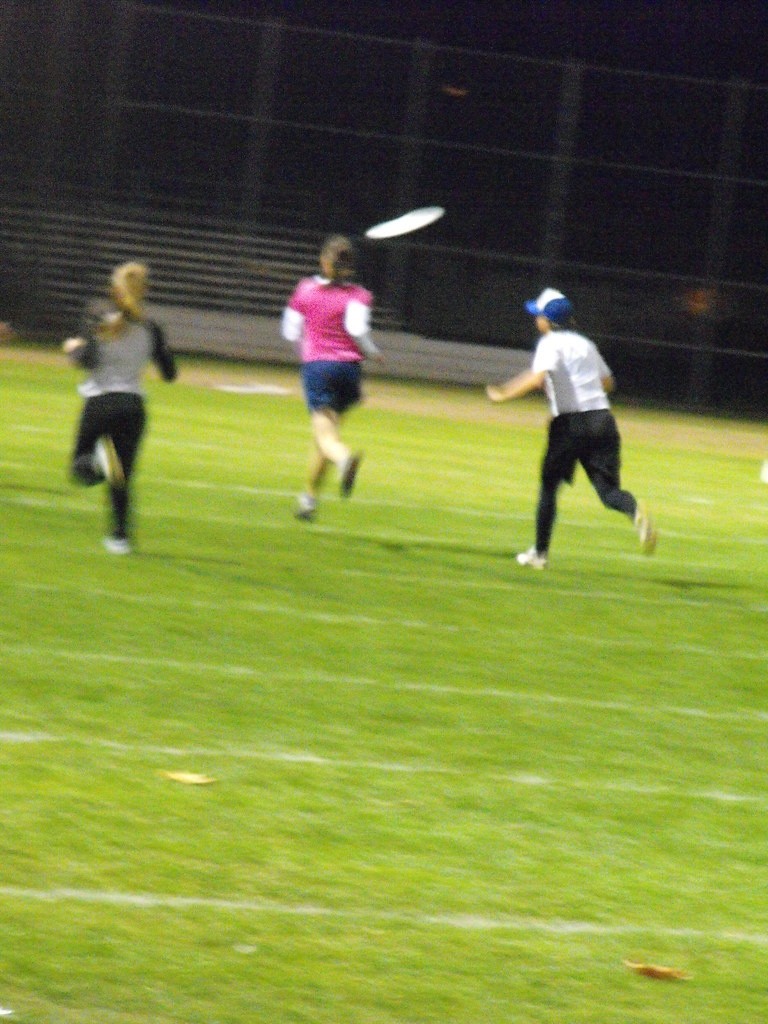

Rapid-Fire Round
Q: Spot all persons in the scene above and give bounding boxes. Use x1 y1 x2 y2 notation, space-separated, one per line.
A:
484 288 659 573
62 262 176 557
283 234 384 522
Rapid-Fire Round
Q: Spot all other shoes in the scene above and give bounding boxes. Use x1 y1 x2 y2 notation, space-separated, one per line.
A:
95 434 125 490
103 536 129 555
516 547 549 570
295 494 315 518
637 516 656 553
335 452 359 497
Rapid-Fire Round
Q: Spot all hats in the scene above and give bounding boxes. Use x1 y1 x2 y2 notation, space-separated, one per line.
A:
525 287 572 324
